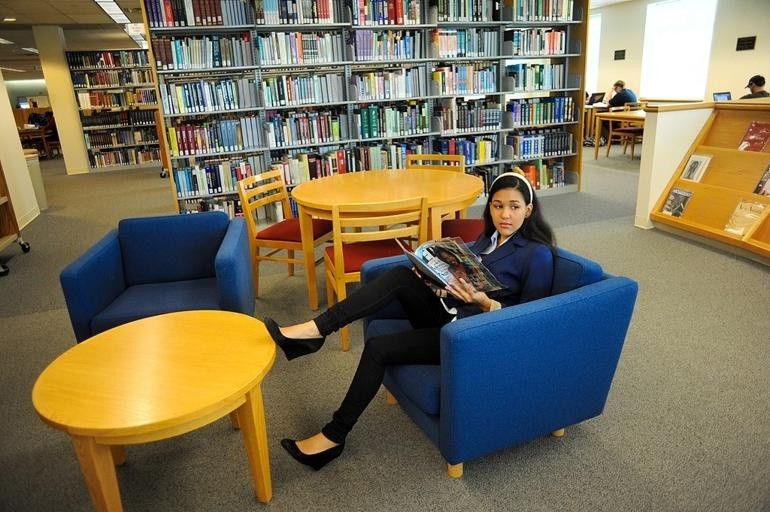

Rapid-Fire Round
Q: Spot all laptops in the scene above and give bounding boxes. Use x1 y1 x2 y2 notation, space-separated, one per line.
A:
713 92 731 102
586 92 605 105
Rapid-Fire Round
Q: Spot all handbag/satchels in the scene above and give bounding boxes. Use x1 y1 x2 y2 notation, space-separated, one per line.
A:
582 134 607 148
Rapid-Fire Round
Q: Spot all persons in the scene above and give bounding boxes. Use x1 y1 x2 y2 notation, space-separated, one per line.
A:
598 80 639 146
263 171 560 472
740 75 770 99
433 246 486 291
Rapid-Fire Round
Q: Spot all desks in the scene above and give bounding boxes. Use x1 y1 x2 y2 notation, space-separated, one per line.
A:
594 109 646 161
19 130 53 160
32 310 276 512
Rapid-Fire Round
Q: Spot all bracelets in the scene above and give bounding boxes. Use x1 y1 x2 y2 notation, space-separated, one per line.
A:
434 289 437 295
440 289 442 297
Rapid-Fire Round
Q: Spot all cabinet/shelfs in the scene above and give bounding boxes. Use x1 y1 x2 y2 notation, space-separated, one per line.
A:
648 95 770 267
139 0 591 214
65 48 164 172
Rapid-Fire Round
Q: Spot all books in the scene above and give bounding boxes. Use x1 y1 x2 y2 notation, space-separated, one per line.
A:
248 0 358 218
393 236 509 301
62 47 161 169
681 155 713 184
427 0 500 195
723 198 770 237
736 121 770 152
144 1 266 219
504 1 576 189
661 187 693 219
751 163 770 198
345 0 429 171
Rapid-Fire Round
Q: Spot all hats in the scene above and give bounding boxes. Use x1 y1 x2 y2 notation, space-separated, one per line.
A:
743 74 767 89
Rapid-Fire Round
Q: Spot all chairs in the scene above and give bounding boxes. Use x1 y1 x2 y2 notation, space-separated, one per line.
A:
606 102 648 160
361 241 638 478
46 141 61 159
60 211 256 343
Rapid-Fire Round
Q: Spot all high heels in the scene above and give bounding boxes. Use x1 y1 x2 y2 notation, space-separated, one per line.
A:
261 313 328 362
276 434 346 473
